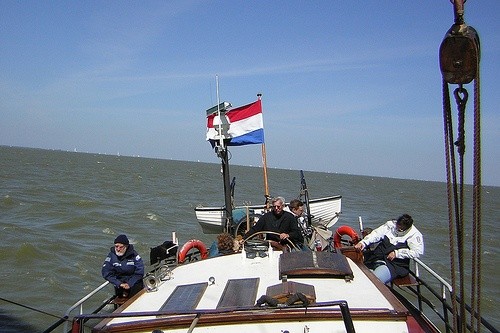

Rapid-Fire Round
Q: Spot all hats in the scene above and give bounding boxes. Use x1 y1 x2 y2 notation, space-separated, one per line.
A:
114 234 129 245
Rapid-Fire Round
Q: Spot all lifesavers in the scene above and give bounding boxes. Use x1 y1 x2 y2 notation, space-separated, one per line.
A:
333 225 359 249
178 239 208 264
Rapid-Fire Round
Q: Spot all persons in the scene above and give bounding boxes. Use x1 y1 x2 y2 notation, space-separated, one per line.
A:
362 227 379 261
353 214 424 285
214 233 237 257
288 199 306 234
238 195 304 251
101 234 144 309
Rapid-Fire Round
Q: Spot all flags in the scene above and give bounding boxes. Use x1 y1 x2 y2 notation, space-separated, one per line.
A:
206 99 265 147
